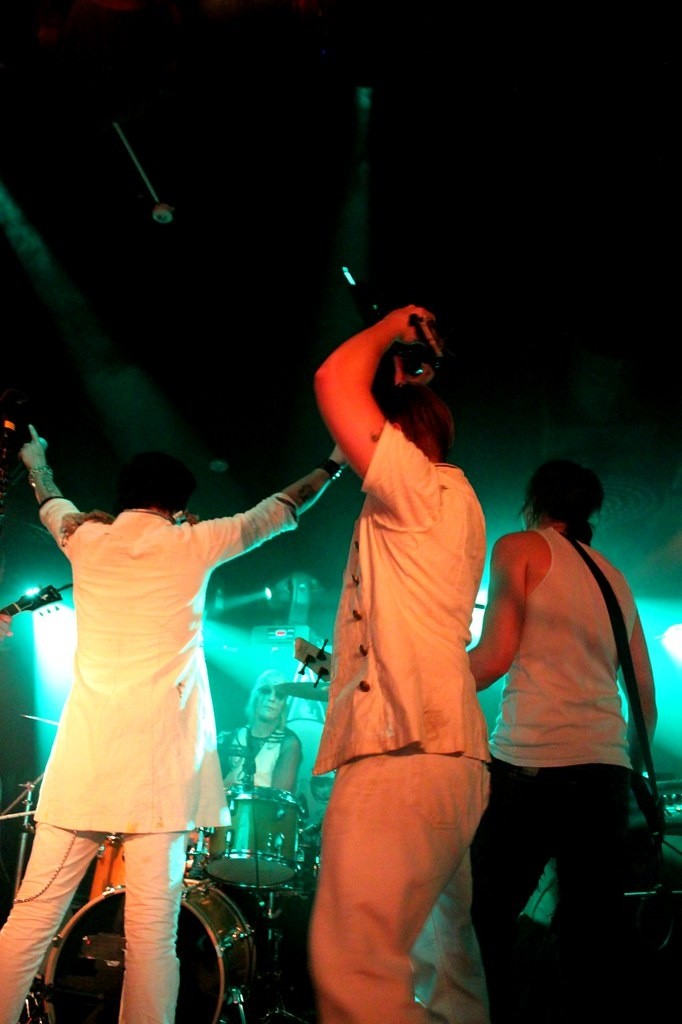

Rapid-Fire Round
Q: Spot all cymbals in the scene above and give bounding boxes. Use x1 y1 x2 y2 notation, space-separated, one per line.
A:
274 681 330 703
19 712 59 727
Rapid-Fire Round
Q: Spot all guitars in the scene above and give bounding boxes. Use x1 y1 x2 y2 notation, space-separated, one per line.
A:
0 581 73 617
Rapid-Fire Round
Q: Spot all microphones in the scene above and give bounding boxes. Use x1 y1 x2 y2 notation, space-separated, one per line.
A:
241 729 257 775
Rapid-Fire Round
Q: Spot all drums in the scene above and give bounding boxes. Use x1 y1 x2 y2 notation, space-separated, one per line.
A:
201 784 299 890
39 878 255 1024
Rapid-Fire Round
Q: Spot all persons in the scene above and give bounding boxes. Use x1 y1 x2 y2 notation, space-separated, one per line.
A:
419 440 656 1022
199 663 303 860
307 299 499 1024
298 765 343 842
1 394 356 1024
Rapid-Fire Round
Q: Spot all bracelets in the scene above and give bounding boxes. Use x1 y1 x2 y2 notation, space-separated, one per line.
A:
316 451 348 483
25 463 55 491
